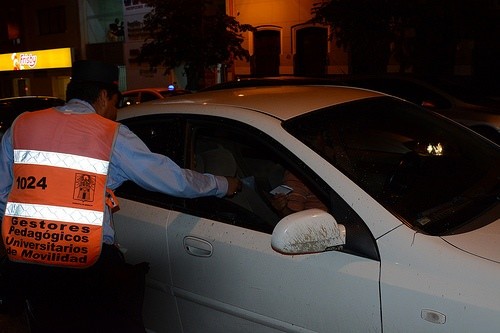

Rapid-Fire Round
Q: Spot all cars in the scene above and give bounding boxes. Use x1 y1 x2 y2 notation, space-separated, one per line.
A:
114 84 500 333
0 76 500 148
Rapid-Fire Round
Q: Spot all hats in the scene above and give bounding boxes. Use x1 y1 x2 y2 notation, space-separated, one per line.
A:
66 61 120 88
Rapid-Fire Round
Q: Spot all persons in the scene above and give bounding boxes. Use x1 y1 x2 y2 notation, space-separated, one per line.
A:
0 79 243 333
273 171 328 212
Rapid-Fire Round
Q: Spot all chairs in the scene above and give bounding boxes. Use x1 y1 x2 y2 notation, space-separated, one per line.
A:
194 141 286 226
